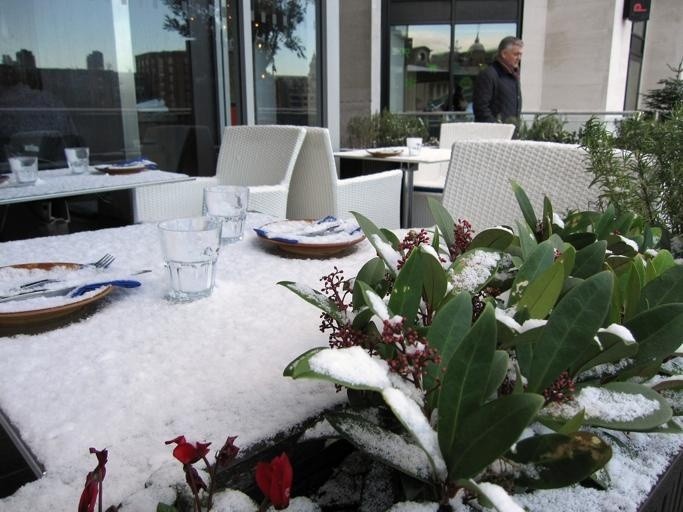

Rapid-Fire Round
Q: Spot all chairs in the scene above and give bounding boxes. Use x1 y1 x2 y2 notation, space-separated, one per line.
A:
284 127 404 233
407 121 516 233
124 124 307 232
435 136 681 258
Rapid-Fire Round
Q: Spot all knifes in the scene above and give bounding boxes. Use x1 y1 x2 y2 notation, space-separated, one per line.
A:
2 269 152 303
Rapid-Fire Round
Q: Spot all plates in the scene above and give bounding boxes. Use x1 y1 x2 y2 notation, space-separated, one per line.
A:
97 163 151 174
369 150 405 156
260 219 369 257
0 173 9 185
2 264 116 329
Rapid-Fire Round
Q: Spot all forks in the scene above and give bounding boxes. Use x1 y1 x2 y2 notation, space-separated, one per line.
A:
1 250 115 298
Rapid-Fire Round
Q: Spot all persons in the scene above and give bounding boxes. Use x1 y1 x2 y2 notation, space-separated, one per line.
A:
0 63 79 161
442 83 466 113
472 35 522 123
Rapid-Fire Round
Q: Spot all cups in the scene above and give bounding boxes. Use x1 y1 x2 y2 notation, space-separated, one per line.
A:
156 218 222 302
406 138 424 156
10 156 39 187
199 186 251 244
61 147 90 174
